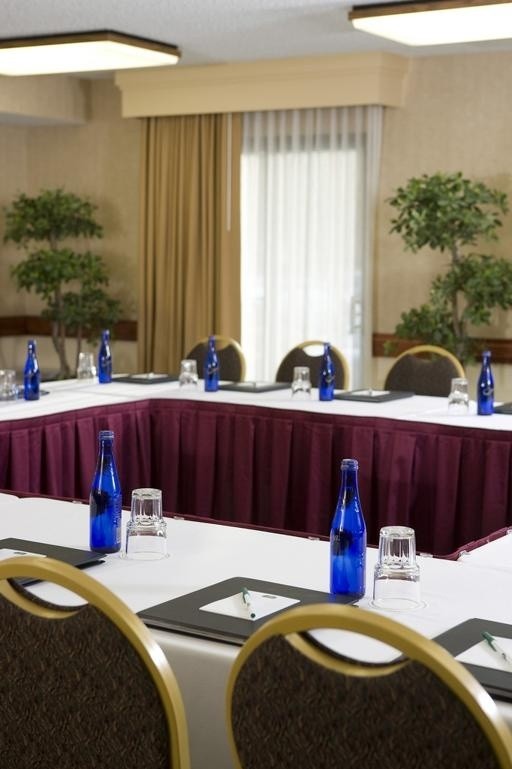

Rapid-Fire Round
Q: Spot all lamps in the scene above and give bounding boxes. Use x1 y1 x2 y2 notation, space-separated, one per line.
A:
0 28 179 76
348 0 511 47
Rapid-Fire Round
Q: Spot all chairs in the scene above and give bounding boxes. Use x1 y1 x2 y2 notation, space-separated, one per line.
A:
223 601 512 769
274 339 350 389
382 343 466 395
186 336 245 381
0 556 193 769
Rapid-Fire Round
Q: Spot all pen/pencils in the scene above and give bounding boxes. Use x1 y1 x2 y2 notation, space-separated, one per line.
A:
243 587 256 618
481 631 512 666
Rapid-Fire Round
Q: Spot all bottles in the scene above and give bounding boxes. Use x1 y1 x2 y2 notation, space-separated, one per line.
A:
88 430 124 555
328 457 368 600
476 351 496 415
203 335 220 392
22 337 41 401
98 329 113 384
319 342 336 401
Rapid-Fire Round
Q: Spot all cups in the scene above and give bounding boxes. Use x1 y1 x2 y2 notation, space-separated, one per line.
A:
123 488 170 560
446 377 470 416
76 351 98 381
290 366 313 402
178 358 199 394
371 526 423 613
0 369 19 403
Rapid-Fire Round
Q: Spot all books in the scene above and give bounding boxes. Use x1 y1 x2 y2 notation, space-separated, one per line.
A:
334 388 413 402
134 576 359 648
0 537 106 590
494 401 512 414
113 373 179 384
219 380 291 393
392 617 512 701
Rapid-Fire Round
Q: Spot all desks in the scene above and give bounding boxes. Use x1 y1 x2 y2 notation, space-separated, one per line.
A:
0 372 512 769
